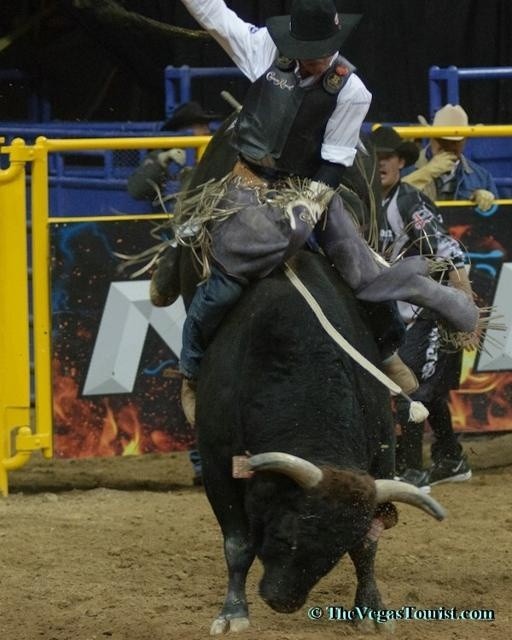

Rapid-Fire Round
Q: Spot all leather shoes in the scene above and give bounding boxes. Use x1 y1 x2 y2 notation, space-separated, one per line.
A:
181 379 195 426
383 352 419 395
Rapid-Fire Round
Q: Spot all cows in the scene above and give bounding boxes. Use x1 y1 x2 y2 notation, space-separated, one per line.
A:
150 98 447 637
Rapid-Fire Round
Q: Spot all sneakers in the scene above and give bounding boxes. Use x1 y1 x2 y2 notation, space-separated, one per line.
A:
429 455 473 487
391 468 431 494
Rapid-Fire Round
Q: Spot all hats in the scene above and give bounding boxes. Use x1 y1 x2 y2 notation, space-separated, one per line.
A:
419 103 484 141
161 101 219 133
372 125 420 168
266 0 365 60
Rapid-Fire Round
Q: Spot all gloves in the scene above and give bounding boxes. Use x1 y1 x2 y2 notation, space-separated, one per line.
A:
401 150 459 191
471 189 495 212
159 149 186 169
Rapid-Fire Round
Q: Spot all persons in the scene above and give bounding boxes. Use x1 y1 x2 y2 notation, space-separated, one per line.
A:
124 100 220 226
401 103 500 219
173 1 420 429
48 207 512 456
372 120 472 487
